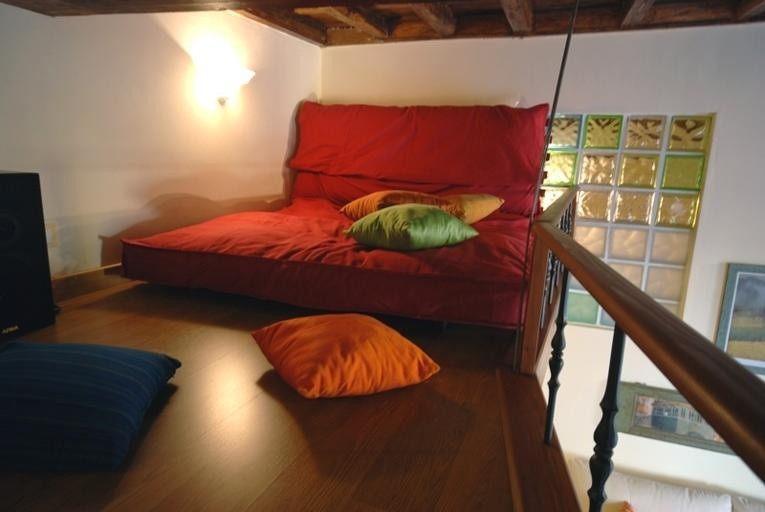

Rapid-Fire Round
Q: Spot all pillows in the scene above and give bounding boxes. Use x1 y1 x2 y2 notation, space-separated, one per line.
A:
338 190 466 222
251 314 440 399
439 192 505 227
343 204 480 250
0 339 182 477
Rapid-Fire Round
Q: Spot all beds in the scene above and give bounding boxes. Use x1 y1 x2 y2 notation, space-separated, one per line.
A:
119 101 551 337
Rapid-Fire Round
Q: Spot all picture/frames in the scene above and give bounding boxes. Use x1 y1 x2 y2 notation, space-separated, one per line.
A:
715 261 765 378
